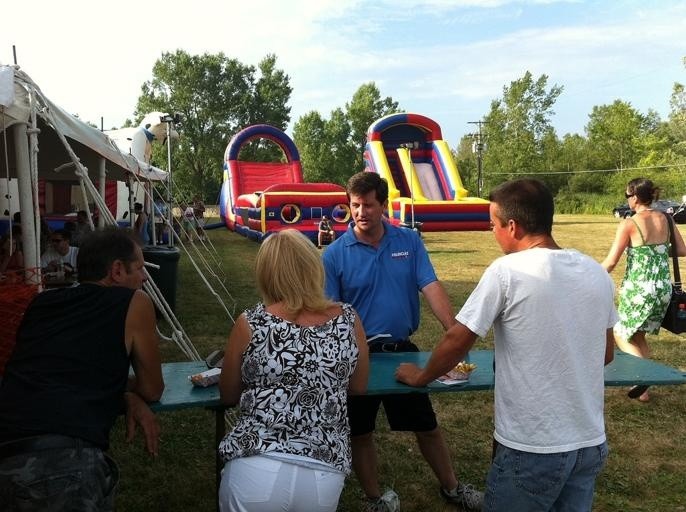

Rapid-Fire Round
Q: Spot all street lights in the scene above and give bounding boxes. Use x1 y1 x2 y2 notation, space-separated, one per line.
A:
159 114 181 249
476 142 483 197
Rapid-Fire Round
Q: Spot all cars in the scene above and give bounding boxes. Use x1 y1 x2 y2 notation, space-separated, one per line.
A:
623 199 686 225
611 200 628 219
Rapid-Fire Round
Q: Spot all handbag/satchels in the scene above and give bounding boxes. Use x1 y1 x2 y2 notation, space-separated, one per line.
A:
661 284 686 335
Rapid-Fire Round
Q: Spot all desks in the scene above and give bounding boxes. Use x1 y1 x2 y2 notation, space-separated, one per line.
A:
44 271 77 290
128 350 686 511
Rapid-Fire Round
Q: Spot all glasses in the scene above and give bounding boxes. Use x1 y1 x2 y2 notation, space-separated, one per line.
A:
52 238 64 243
625 193 636 198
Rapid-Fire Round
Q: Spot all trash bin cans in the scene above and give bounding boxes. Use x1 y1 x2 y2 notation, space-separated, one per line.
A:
141 246 181 319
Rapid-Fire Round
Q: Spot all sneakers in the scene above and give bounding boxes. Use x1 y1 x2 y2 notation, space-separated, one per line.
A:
152 237 207 245
440 480 485 510
366 491 403 512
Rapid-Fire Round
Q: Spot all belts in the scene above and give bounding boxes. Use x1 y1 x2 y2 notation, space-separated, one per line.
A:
0 435 107 458
367 342 416 353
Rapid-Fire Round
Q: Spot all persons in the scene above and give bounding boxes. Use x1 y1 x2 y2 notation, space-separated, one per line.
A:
9 196 207 285
217 228 370 512
1 228 163 512
318 171 484 509
396 180 619 511
318 216 336 249
601 178 686 401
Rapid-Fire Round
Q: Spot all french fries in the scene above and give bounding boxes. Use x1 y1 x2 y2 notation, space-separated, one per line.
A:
191 374 203 381
455 360 477 372
